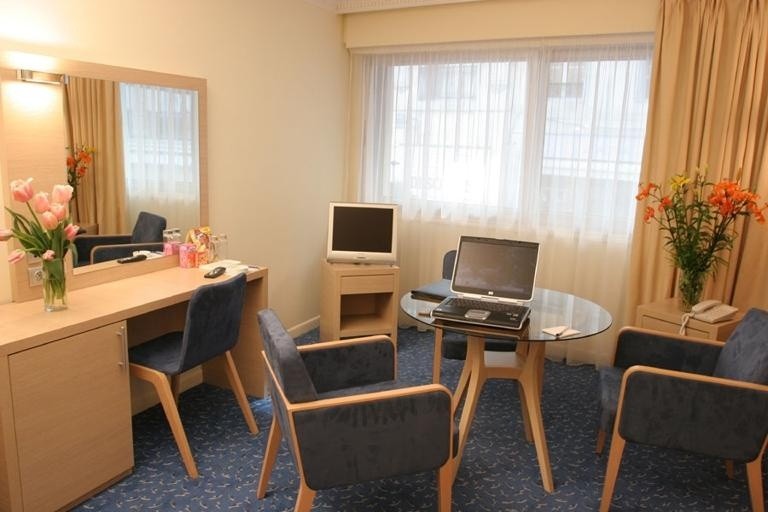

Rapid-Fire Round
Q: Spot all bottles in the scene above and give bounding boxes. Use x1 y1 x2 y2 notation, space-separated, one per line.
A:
212 236 221 261
173 229 182 242
163 230 173 255
220 234 227 260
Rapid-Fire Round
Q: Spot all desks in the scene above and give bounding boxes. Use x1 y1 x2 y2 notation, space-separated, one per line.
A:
0 257 269 512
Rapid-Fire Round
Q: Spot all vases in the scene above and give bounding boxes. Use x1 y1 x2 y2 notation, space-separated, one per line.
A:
677 266 707 312
41 258 68 313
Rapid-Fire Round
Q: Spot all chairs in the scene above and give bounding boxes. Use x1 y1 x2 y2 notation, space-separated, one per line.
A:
595 308 768 512
256 308 459 512
432 250 545 406
128 272 259 478
73 211 166 267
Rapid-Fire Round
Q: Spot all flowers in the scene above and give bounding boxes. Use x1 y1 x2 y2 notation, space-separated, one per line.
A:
635 161 768 308
0 178 74 303
65 143 96 222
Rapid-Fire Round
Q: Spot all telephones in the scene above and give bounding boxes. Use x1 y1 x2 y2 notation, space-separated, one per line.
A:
691 300 739 324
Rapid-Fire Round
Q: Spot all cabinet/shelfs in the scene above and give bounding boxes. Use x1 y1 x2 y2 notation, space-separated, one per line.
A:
319 258 400 383
70 222 97 237
635 296 746 343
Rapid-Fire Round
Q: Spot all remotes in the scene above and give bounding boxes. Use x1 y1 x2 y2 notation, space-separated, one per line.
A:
116 254 147 264
205 266 225 278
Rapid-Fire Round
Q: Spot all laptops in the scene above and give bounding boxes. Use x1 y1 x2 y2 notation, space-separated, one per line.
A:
431 316 531 341
431 236 541 331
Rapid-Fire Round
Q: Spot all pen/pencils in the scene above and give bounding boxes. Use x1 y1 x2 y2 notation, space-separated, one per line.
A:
557 327 571 336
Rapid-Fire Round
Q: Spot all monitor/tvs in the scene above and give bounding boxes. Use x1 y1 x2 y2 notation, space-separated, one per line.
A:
326 202 400 264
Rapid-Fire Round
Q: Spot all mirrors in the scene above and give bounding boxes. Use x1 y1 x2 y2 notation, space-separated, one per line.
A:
61 74 200 268
